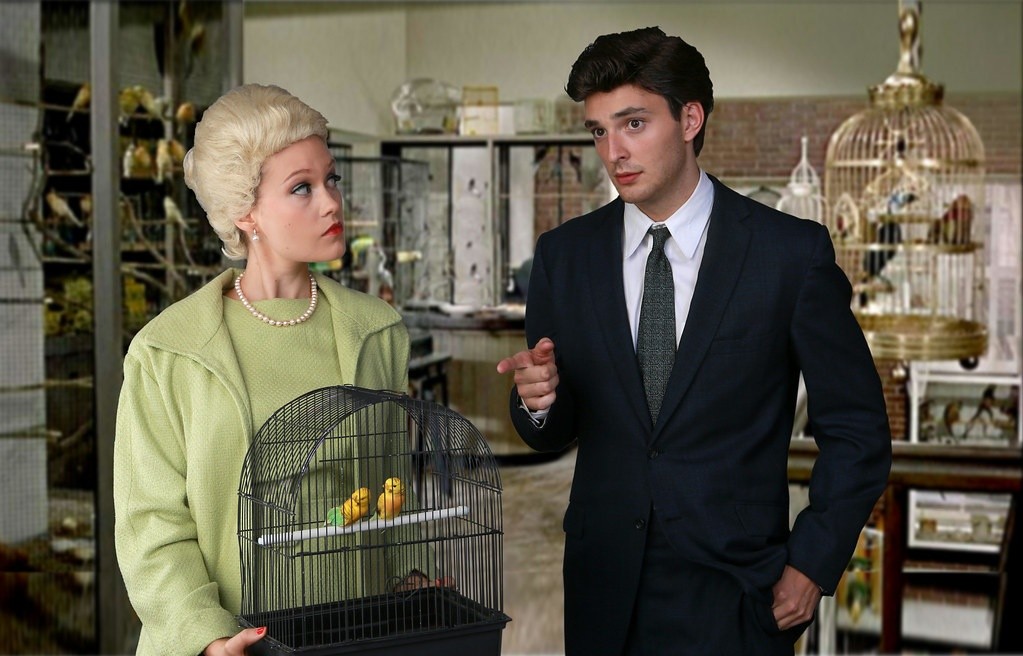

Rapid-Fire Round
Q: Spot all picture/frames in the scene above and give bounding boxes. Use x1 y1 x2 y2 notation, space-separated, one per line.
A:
908 355 1023 448
718 177 823 222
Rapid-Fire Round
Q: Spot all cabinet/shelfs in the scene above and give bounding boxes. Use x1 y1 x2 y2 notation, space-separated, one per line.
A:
328 131 593 467
788 435 1023 656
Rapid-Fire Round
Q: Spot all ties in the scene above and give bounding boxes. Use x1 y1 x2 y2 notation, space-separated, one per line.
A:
634 227 677 431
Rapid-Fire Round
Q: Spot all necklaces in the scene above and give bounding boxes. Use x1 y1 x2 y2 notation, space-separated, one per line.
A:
233 270 320 328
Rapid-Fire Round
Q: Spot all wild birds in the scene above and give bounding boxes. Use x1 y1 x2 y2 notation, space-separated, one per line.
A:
324 487 371 527
371 476 406 522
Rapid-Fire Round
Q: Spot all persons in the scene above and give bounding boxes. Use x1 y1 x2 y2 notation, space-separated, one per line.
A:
494 24 895 656
108 80 461 656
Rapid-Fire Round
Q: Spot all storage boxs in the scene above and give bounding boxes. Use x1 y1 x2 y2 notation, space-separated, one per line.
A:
456 104 537 136
